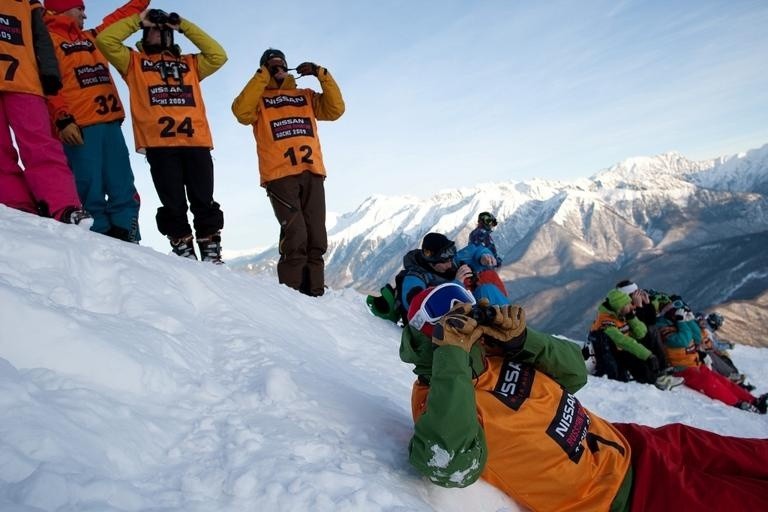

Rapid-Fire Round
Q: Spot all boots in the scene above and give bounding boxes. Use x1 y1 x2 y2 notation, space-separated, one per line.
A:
171 232 198 260
196 229 224 264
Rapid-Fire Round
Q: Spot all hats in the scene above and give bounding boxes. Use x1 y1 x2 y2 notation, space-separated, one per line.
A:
43 0 83 14
650 292 670 312
407 282 477 336
423 233 456 262
607 288 631 310
260 49 284 65
478 212 497 228
617 281 637 294
707 312 723 328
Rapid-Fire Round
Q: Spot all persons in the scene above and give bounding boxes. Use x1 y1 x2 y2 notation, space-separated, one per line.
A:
581 280 768 415
469 212 502 271
396 233 512 313
0 1 94 230
43 0 152 245
399 283 768 512
95 8 228 266
231 48 345 297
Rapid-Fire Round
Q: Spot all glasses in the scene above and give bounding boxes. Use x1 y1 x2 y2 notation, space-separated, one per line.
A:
410 281 476 330
673 300 684 307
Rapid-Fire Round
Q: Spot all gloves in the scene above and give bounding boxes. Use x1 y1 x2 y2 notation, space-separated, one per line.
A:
674 306 694 322
296 62 316 75
432 303 483 352
55 114 83 144
480 297 526 341
650 354 660 371
268 65 287 78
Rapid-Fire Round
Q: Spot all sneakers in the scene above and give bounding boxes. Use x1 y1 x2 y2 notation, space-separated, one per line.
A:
738 395 767 414
71 210 93 226
656 375 685 390
730 373 745 385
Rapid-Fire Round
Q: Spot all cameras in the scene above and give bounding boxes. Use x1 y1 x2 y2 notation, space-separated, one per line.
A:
470 303 497 328
